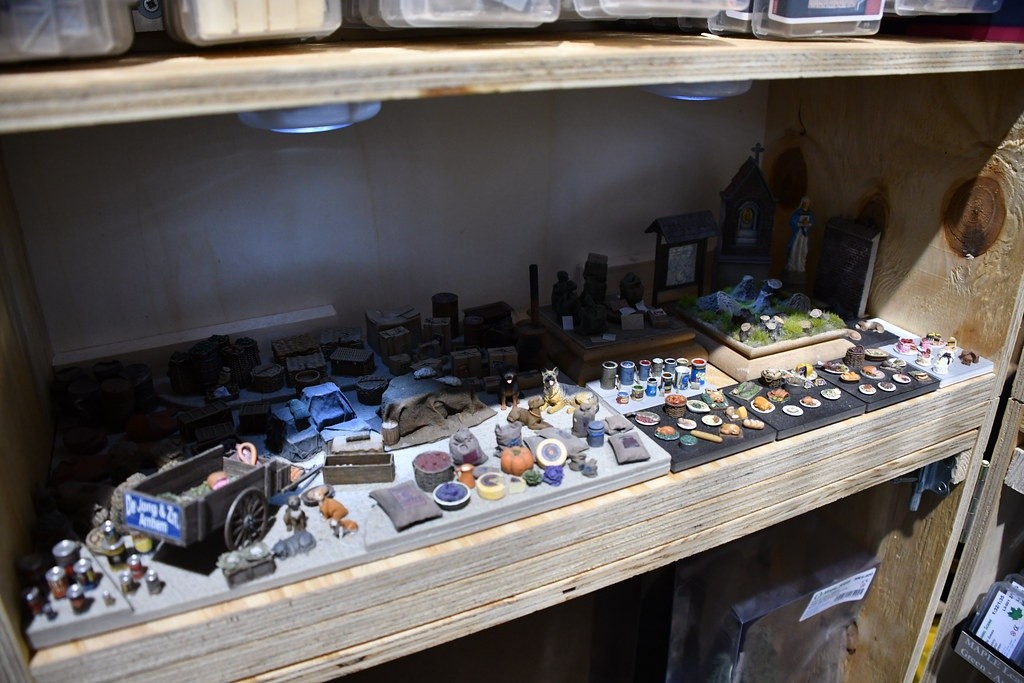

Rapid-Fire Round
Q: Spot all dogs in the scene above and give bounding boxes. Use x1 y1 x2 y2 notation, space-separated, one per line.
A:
500 369 521 410
538 366 599 416
507 398 553 430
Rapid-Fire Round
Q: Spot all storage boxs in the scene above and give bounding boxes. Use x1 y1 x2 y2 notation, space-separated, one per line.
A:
752 0 885 40
906 0 1024 42
558 0 750 19
677 17 708 33
1002 574 1024 594
161 0 346 49
707 0 768 36
935 616 1024 683
358 0 562 30
970 580 1024 668
0 0 135 62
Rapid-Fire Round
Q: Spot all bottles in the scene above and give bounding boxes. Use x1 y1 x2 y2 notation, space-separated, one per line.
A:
587 421 605 446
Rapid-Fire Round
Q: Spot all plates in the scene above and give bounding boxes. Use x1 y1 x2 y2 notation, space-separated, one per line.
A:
702 415 722 426
932 367 948 374
840 374 860 381
782 405 803 416
821 389 841 399
635 412 660 425
751 400 776 414
825 363 850 373
893 374 911 383
702 389 728 409
859 385 876 394
677 419 697 429
800 399 821 407
915 360 930 366
687 400 710 413
432 482 470 506
893 344 916 355
655 431 679 440
878 382 896 391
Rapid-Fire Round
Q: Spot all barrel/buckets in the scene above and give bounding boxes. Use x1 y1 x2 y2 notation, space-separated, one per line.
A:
382 419 399 445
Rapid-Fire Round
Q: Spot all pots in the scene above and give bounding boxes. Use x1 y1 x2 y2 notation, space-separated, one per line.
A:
860 369 885 379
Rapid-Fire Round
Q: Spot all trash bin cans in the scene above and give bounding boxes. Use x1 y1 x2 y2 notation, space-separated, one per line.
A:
935 612 1024 683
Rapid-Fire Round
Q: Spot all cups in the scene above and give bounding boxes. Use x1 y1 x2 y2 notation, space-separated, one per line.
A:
295 370 320 400
600 357 707 404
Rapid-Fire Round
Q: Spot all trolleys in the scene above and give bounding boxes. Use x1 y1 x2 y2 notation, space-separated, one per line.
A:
115 443 324 559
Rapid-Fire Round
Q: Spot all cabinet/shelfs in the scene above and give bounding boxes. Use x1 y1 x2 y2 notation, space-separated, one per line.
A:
0 34 1024 683
